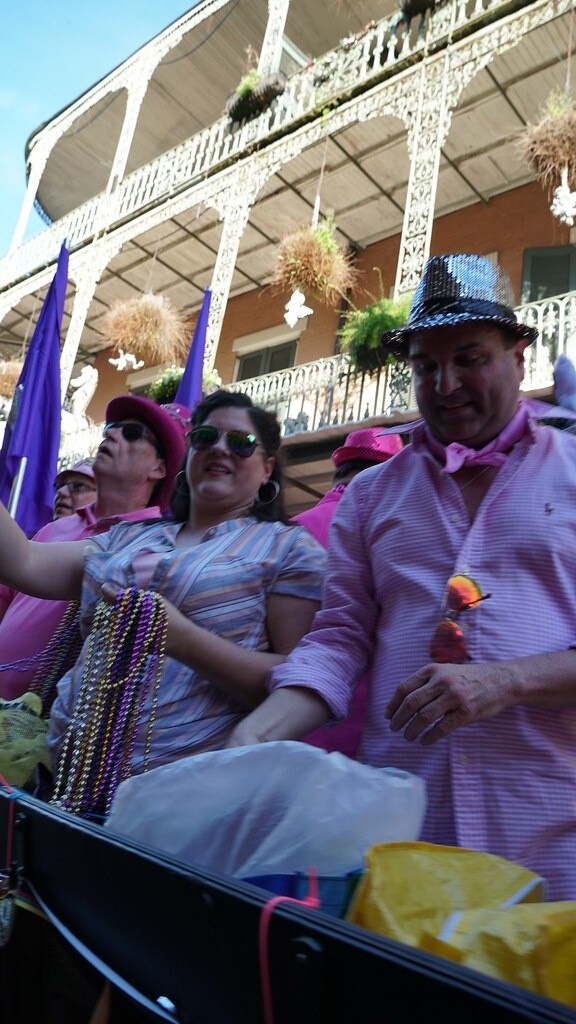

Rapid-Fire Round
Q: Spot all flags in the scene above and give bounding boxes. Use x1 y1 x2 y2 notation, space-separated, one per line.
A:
174 290 211 412
0 244 69 537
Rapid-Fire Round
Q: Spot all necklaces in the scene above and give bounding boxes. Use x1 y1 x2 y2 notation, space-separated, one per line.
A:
46 590 169 820
0 598 84 711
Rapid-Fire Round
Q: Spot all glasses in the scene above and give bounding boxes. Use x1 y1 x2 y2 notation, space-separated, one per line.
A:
430 574 492 663
103 422 162 453
186 425 264 458
54 481 96 492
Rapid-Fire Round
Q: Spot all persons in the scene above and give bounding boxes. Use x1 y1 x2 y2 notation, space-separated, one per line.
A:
0 388 327 830
221 253 576 923
0 396 190 701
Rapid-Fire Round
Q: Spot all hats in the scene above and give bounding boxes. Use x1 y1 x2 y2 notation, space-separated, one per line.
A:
332 428 404 467
381 253 539 360
106 395 193 487
54 456 96 492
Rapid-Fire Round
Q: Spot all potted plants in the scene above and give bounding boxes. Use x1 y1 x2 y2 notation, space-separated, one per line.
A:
222 64 283 120
334 264 411 379
140 365 221 410
